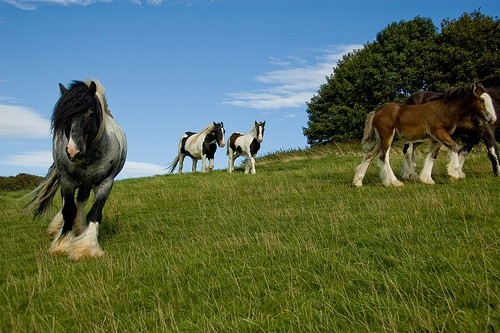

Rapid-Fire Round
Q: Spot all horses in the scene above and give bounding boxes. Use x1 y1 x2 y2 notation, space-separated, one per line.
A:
352 78 498 185
21 79 127 259
161 121 267 173
